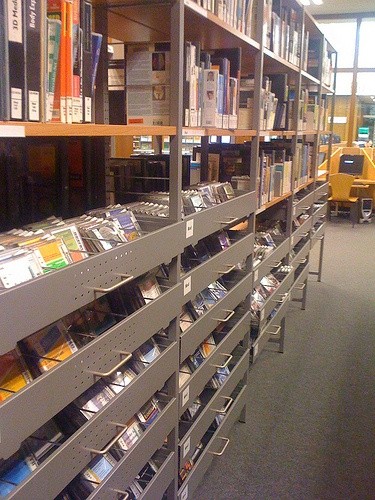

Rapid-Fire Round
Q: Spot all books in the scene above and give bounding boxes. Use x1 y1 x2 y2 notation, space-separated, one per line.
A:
191 0 253 39
108 153 236 217
254 214 310 234
55 393 164 500
119 457 158 500
320 93 328 131
264 0 301 69
288 88 320 130
123 40 243 131
193 141 292 210
308 220 323 235
233 231 277 270
237 264 293 310
178 399 232 489
0 337 162 500
245 292 288 347
154 229 232 277
157 279 229 336
4 0 103 124
244 139 314 189
282 183 313 204
0 137 143 291
297 204 317 225
165 362 231 443
322 39 331 87
303 32 324 79
0 267 163 406
239 72 289 130
158 331 219 395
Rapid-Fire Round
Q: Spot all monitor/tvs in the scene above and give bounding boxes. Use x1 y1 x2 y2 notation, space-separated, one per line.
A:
338 154 364 176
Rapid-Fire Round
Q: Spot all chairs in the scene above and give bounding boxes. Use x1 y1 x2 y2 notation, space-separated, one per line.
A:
328 174 359 228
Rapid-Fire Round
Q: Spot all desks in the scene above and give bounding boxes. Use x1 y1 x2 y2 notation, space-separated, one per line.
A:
317 173 375 220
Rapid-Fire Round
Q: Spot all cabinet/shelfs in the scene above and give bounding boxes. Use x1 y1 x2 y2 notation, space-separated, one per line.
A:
1 0 339 500
